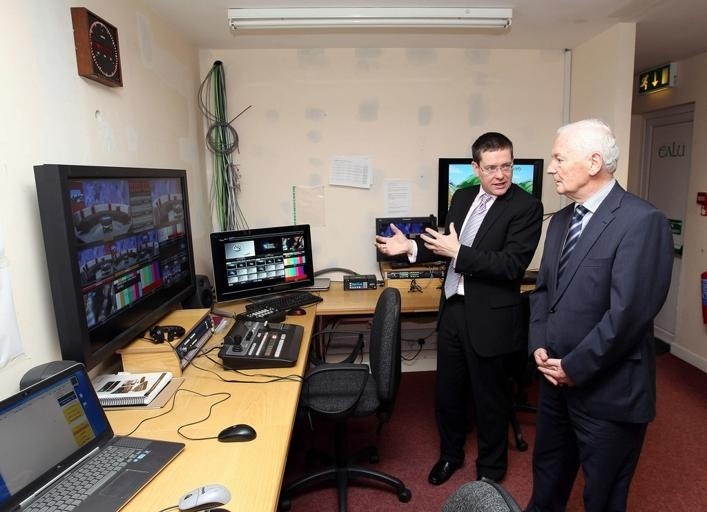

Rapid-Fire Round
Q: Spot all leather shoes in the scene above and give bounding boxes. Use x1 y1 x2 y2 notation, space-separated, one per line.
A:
429 459 463 485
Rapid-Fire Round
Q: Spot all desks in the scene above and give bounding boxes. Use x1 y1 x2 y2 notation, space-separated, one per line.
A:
81 281 318 511
317 278 536 376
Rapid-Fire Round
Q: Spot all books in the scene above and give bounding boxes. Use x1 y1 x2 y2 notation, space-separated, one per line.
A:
88 371 173 405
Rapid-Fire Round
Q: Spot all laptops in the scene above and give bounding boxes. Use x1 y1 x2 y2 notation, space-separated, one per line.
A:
1 362 186 511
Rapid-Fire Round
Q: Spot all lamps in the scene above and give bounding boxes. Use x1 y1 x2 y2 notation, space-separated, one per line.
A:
225 8 512 34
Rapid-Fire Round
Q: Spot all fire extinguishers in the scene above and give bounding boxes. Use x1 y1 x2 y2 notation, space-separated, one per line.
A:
701 271 707 326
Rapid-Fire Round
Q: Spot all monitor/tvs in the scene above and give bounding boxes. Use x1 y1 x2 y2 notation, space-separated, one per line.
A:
437 158 544 228
32 163 197 375
375 217 438 269
209 225 314 304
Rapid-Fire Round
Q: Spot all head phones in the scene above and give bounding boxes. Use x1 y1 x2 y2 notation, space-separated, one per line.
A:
149 324 186 344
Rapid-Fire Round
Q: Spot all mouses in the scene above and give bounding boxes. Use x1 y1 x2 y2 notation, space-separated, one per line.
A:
177 483 233 512
287 308 306 315
287 306 306 317
218 424 257 443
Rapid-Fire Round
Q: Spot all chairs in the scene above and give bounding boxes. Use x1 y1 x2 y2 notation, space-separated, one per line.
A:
437 477 521 512
280 288 412 512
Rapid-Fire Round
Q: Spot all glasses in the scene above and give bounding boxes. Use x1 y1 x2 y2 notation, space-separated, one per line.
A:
481 162 513 175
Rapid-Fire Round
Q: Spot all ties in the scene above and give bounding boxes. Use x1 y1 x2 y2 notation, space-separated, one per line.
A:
445 195 490 299
557 205 588 287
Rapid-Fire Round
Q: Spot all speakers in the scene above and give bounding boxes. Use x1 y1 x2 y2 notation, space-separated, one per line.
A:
181 273 213 309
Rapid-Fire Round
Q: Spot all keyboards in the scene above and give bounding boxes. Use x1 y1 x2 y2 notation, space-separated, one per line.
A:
244 291 324 317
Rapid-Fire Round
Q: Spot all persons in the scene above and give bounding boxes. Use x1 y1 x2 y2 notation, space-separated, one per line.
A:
372 132 542 486
526 120 673 512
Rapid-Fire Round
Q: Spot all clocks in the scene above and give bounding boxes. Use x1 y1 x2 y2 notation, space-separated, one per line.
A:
69 8 124 89
